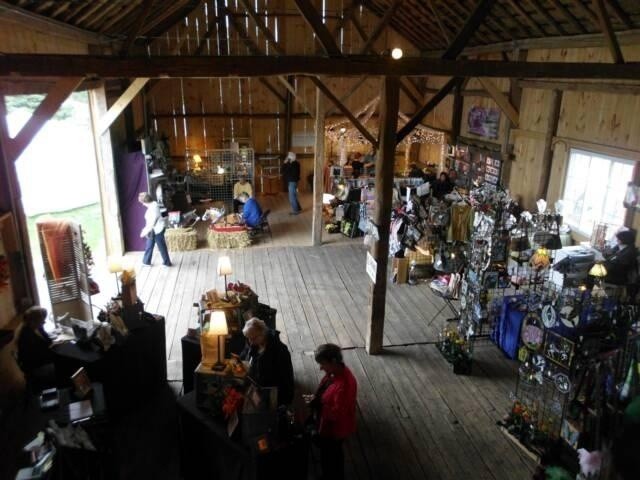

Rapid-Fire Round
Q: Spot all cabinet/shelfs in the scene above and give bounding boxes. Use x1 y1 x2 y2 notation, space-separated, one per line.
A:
259 155 281 193
340 186 362 238
551 245 606 295
393 257 409 285
507 215 564 295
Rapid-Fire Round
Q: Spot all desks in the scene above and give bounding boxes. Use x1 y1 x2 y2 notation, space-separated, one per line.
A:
491 295 626 360
155 228 198 251
207 228 251 250
429 278 463 326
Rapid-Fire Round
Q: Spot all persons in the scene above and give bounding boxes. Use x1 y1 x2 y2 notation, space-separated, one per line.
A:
605 232 635 266
405 160 458 197
232 317 293 409
14 305 60 386
139 191 173 266
324 152 377 179
304 344 357 478
279 151 302 216
233 175 252 213
238 192 262 231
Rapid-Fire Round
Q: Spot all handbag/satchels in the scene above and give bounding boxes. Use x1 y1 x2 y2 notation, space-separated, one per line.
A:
145 228 155 240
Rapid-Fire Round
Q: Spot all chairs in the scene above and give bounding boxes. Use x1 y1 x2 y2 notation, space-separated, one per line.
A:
248 209 272 243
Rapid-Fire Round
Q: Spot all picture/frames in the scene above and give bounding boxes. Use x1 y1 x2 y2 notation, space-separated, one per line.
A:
543 330 575 369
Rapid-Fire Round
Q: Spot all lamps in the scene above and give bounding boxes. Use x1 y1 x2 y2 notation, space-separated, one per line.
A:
218 256 234 303
589 262 607 288
528 246 550 292
107 252 123 300
